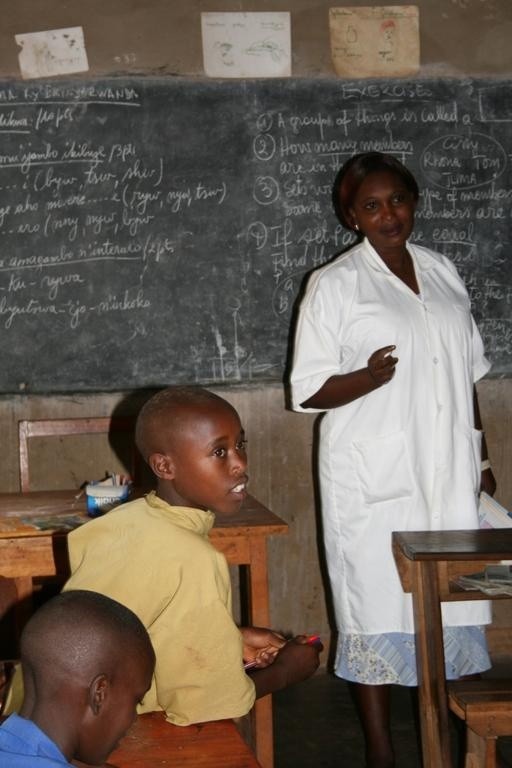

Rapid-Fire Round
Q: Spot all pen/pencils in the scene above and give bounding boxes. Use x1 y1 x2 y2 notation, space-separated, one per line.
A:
240 633 321 669
112 469 129 486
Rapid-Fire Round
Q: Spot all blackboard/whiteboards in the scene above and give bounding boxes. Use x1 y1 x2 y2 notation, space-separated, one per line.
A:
0 74 512 395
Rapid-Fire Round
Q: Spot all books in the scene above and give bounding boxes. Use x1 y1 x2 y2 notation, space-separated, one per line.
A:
478 490 512 528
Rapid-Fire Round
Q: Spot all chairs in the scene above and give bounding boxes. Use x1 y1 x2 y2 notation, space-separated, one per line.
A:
16 418 110 600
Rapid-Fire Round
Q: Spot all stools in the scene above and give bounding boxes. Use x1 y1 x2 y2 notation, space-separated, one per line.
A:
447 677 512 768
107 710 262 768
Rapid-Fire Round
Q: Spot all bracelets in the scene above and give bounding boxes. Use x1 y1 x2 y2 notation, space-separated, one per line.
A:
482 459 492 472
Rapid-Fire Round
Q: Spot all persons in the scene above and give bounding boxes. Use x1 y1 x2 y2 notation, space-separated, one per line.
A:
288 152 497 768
1 590 157 768
4 386 322 726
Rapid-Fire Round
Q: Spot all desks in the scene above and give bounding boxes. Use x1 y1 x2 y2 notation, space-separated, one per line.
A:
391 528 512 768
0 490 288 767
